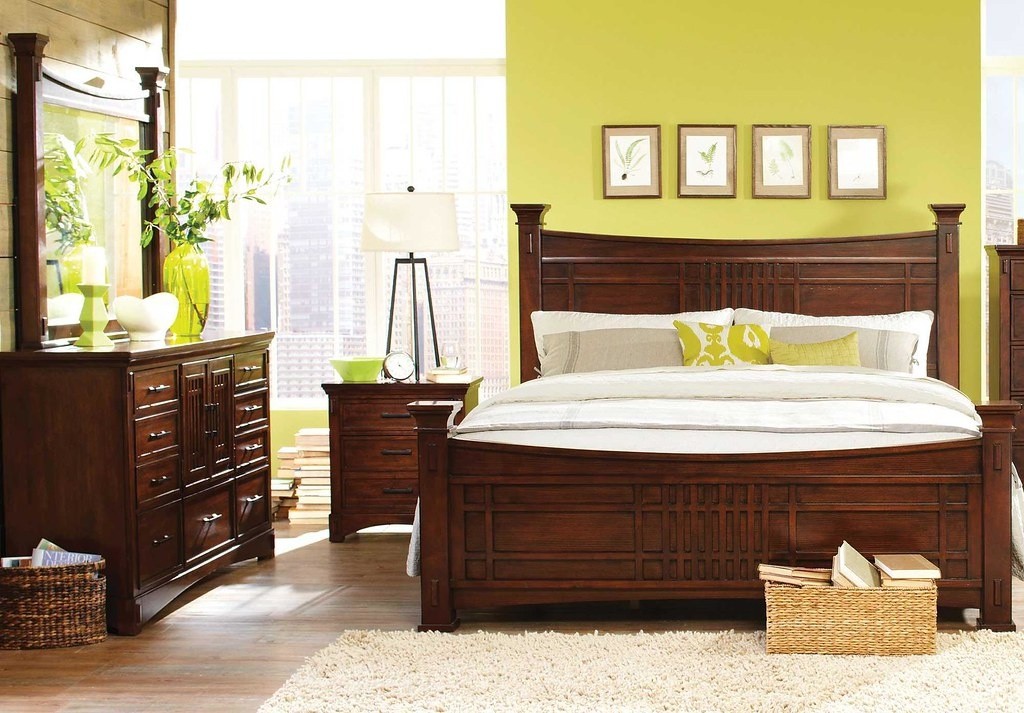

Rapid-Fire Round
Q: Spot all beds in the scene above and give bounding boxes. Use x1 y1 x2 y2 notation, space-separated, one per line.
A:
405 202 1024 632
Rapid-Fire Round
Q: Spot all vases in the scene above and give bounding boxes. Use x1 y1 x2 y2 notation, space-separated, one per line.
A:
74 284 114 347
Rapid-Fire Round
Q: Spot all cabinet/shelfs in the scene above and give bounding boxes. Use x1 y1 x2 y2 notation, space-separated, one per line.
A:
320 375 484 543
994 244 1024 488
0 332 274 636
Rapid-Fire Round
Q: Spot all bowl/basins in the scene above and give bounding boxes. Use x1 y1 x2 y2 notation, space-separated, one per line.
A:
329 359 387 383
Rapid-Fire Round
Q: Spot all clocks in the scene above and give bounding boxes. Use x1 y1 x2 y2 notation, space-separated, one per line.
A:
383 352 414 384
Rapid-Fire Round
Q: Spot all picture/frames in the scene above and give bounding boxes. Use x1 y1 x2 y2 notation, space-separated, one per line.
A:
677 124 737 198
752 124 811 199
602 125 661 198
827 125 888 199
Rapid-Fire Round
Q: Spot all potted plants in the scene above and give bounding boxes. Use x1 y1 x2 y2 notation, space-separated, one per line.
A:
95 133 293 338
44 135 107 303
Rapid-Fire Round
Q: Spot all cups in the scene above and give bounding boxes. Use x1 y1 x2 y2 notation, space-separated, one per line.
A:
439 341 461 370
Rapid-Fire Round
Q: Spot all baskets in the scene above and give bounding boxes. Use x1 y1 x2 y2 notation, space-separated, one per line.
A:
0 558 110 650
764 580 938 656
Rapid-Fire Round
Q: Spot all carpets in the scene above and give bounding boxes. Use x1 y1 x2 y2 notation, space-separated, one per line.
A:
256 627 1024 713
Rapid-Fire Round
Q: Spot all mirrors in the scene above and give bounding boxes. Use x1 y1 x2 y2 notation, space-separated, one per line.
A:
8 31 170 348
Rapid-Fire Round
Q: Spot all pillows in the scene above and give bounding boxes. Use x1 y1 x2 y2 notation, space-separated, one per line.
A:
732 308 934 376
542 327 684 378
673 320 769 367
530 308 734 369
771 326 920 374
769 331 860 367
